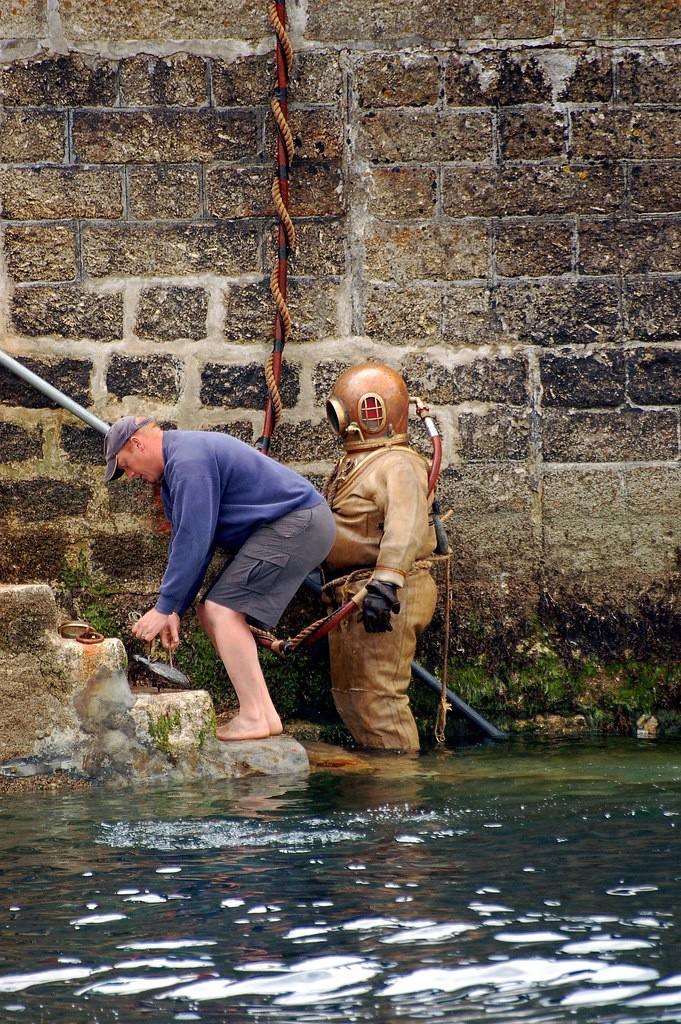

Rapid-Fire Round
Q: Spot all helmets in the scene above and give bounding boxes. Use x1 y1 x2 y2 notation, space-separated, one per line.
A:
325 363 411 451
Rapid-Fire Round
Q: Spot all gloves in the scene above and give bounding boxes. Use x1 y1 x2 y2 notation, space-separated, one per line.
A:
362 583 397 633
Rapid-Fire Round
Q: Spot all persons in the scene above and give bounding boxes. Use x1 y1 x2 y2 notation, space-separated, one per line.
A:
102 414 337 740
320 362 437 750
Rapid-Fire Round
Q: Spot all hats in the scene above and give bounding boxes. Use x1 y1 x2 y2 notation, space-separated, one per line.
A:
103 416 153 480
